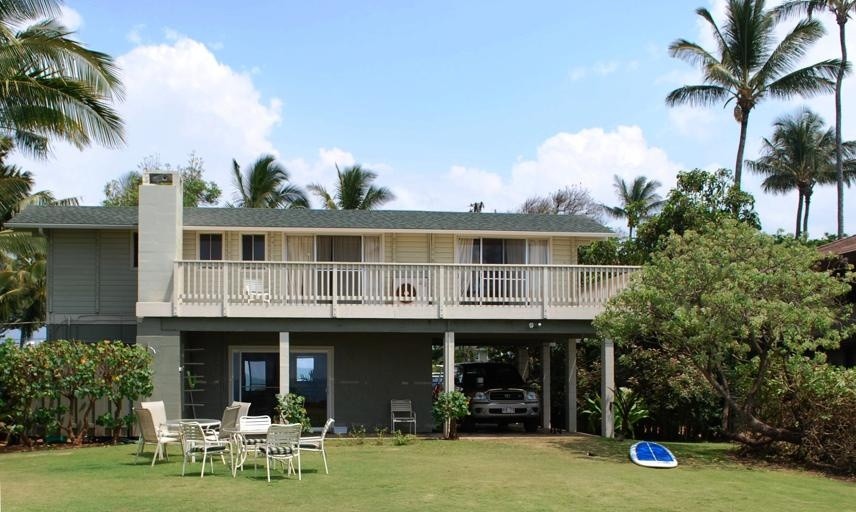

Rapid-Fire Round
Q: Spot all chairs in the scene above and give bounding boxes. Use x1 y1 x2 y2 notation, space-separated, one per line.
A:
242 280 270 304
132 400 336 482
390 399 416 435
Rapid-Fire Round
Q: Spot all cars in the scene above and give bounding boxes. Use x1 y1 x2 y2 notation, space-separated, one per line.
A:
453 360 542 432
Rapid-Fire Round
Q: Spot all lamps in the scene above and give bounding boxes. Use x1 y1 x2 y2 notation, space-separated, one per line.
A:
529 321 542 329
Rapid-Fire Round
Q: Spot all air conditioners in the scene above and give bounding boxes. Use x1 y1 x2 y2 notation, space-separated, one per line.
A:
393 278 428 305
